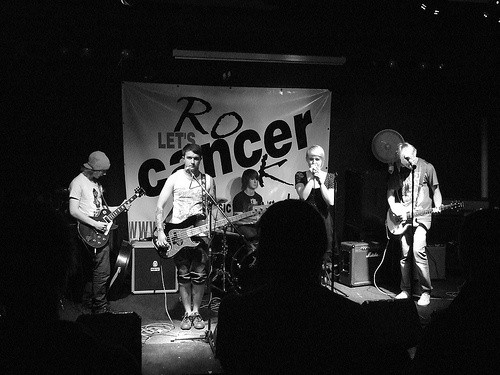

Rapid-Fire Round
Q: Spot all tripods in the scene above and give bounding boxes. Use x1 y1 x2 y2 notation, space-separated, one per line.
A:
212 212 255 297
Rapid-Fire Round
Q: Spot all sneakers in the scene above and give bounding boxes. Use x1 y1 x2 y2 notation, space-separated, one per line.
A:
193 312 205 329
180 312 193 330
417 292 430 306
395 290 409 299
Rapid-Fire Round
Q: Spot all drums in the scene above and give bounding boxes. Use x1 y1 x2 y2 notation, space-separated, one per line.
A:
233 219 259 238
231 241 260 294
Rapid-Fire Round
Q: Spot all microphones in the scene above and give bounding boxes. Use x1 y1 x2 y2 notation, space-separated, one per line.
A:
186 167 190 173
312 169 315 175
404 155 416 168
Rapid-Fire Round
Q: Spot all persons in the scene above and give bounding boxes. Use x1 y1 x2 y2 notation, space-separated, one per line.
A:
233 169 264 260
68 150 132 315
216 198 364 375
155 143 216 330
415 207 500 375
386 142 442 306
295 144 335 262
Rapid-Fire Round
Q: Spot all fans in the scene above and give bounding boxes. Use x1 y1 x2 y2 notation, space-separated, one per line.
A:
370 129 407 164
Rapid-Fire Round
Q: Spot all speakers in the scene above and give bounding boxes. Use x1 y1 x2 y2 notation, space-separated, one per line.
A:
132 246 179 294
339 241 371 287
426 245 446 279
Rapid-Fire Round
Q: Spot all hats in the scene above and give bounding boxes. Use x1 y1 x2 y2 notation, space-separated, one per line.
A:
83 151 110 171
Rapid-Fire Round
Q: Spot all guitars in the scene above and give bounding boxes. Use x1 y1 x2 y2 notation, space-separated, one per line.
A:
385 200 465 235
75 186 146 250
152 199 275 260
